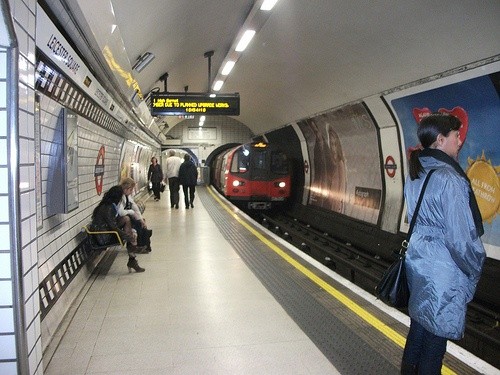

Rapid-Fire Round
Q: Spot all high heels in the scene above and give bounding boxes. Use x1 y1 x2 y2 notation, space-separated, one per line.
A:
127 257 145 273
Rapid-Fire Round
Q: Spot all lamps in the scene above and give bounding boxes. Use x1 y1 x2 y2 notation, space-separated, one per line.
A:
131 51 155 72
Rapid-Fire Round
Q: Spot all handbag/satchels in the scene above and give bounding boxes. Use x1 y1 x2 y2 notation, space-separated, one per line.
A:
374 254 411 308
148 181 153 190
160 182 165 192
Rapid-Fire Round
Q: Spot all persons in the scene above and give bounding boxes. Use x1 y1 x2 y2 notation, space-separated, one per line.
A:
117 179 152 252
180 154 198 208
400 113 487 375
164 150 183 209
91 184 145 272
148 157 164 200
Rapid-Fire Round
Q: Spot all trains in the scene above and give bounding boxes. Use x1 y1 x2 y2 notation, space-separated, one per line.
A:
211 140 292 202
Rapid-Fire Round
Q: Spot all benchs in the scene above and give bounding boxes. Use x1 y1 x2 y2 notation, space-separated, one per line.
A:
82 223 127 250
